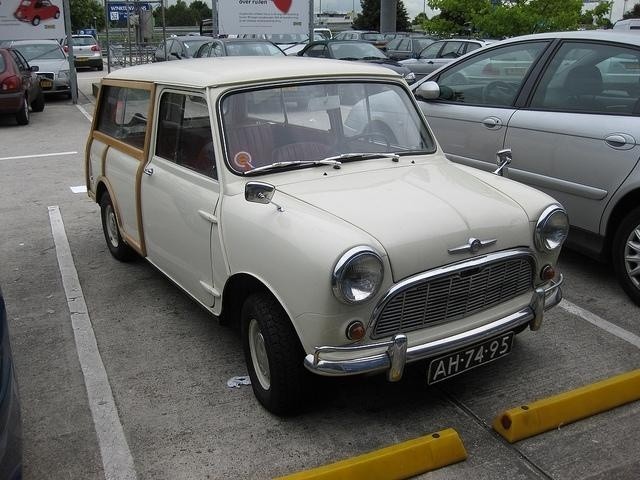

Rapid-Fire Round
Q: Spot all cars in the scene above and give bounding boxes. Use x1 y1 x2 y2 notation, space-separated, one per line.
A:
81 57 571 417
0 48 44 125
8 38 78 103
14 0 61 26
346 31 640 312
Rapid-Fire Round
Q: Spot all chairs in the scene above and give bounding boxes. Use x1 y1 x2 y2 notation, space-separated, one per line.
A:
226 124 277 169
273 142 338 161
562 65 608 111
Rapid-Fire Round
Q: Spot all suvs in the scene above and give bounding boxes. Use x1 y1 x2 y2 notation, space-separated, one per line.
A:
61 35 104 72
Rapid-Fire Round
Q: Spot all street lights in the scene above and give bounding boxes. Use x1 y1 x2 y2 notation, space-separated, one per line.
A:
94 17 97 28
